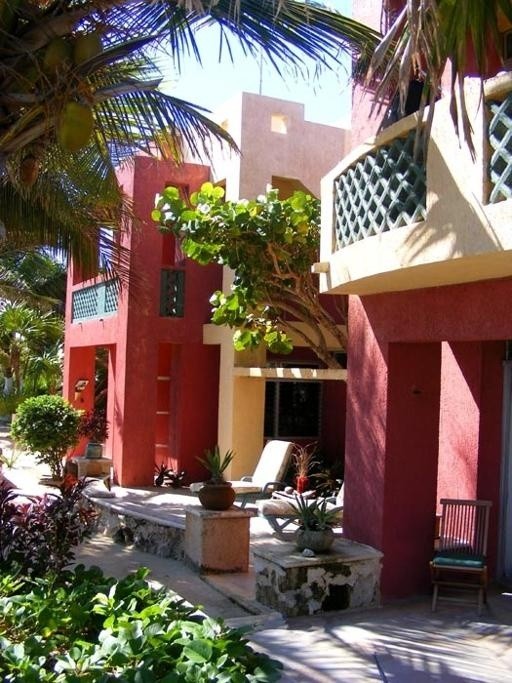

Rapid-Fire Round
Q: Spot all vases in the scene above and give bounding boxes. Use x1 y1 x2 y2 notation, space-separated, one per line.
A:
296 477 310 493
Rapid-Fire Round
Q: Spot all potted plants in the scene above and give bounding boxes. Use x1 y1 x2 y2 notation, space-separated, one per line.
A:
195 445 236 511
287 492 338 553
80 409 109 459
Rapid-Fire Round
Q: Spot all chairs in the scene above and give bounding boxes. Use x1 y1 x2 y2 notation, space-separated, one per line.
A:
256 481 344 541
190 439 294 510
429 499 493 616
